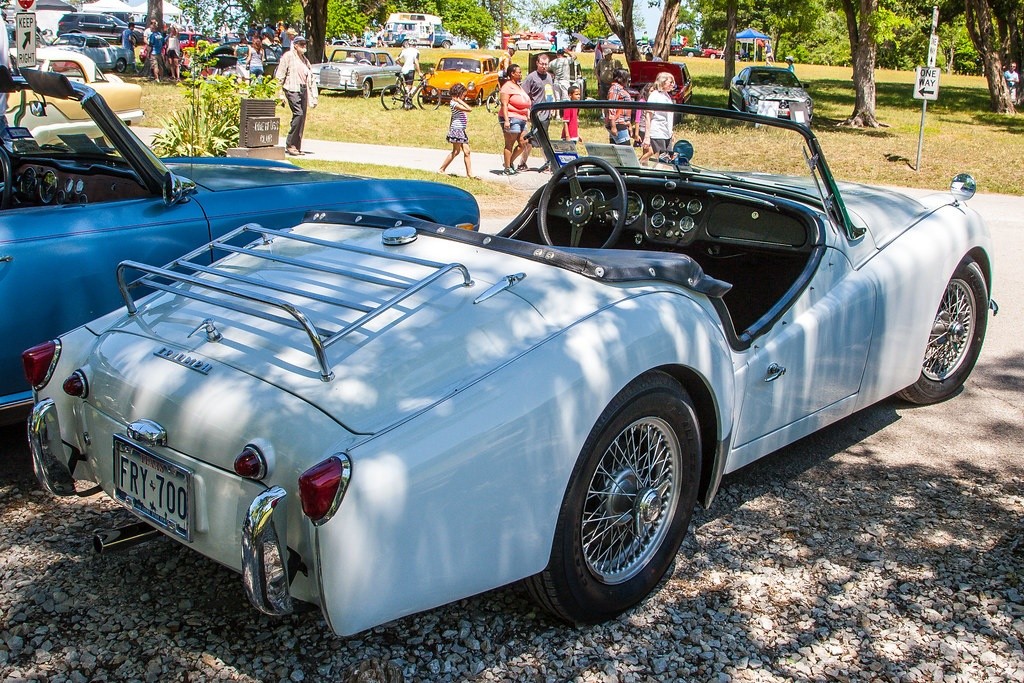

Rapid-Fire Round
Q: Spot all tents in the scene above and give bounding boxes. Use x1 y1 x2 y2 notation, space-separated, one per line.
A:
722 28 772 61
0 0 185 20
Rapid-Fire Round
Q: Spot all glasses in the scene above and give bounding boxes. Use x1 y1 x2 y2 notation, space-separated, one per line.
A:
295 42 307 45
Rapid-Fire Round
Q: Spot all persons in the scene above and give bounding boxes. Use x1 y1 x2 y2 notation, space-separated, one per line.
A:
635 72 675 164
605 68 633 146
498 43 517 90
561 86 581 145
395 38 423 110
121 21 181 83
738 41 794 73
1003 62 1019 103
275 35 319 156
469 30 684 61
336 20 434 50
174 17 298 94
545 48 623 119
128 12 134 22
0 15 10 133
498 64 531 175
521 54 555 174
437 84 479 179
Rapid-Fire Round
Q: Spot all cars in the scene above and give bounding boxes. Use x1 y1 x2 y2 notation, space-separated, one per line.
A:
0 0 284 151
324 12 455 49
0 63 481 432
309 46 404 100
421 51 503 107
502 32 725 61
526 48 587 117
727 64 814 131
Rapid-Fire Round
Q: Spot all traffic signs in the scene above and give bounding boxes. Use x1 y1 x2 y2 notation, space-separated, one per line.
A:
15 11 37 68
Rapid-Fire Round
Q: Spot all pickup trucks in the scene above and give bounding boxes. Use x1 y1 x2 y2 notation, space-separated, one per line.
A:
622 57 693 125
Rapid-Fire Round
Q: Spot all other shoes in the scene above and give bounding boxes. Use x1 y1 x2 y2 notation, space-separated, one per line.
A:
517 163 529 172
503 163 517 170
503 168 515 175
286 145 305 156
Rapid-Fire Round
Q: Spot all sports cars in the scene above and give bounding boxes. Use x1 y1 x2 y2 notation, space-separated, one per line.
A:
17 97 1001 650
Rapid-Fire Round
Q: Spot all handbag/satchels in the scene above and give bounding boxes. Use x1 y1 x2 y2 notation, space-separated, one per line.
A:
307 71 318 109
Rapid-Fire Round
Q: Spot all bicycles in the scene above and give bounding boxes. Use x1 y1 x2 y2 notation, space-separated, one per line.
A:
380 71 442 111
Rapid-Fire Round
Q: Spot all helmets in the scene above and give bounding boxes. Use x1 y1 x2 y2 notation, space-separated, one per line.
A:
408 37 418 45
507 44 517 50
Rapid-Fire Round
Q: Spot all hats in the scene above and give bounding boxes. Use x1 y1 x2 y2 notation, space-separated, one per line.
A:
785 56 793 62
293 35 308 42
604 49 613 57
